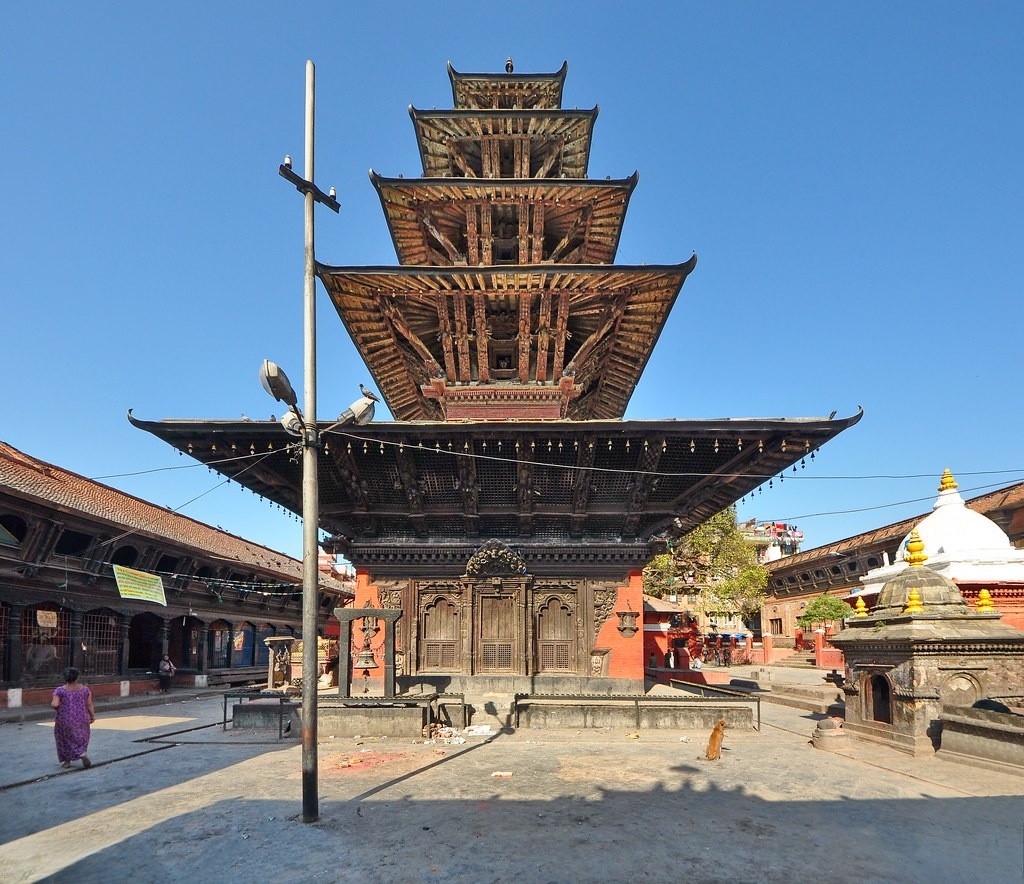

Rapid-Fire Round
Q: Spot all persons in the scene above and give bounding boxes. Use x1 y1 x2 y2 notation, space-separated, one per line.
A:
276 647 290 679
712 645 721 666
702 644 708 663
159 654 176 694
723 646 731 667
51 668 96 768
650 652 657 681
665 649 671 668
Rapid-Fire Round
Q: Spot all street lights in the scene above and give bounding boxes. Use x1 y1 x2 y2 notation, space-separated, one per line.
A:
259 57 378 823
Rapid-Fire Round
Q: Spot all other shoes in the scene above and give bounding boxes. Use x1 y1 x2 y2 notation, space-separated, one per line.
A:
80 755 91 769
61 762 71 769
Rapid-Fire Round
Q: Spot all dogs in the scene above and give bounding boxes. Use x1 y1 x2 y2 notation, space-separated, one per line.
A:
697 720 735 760
